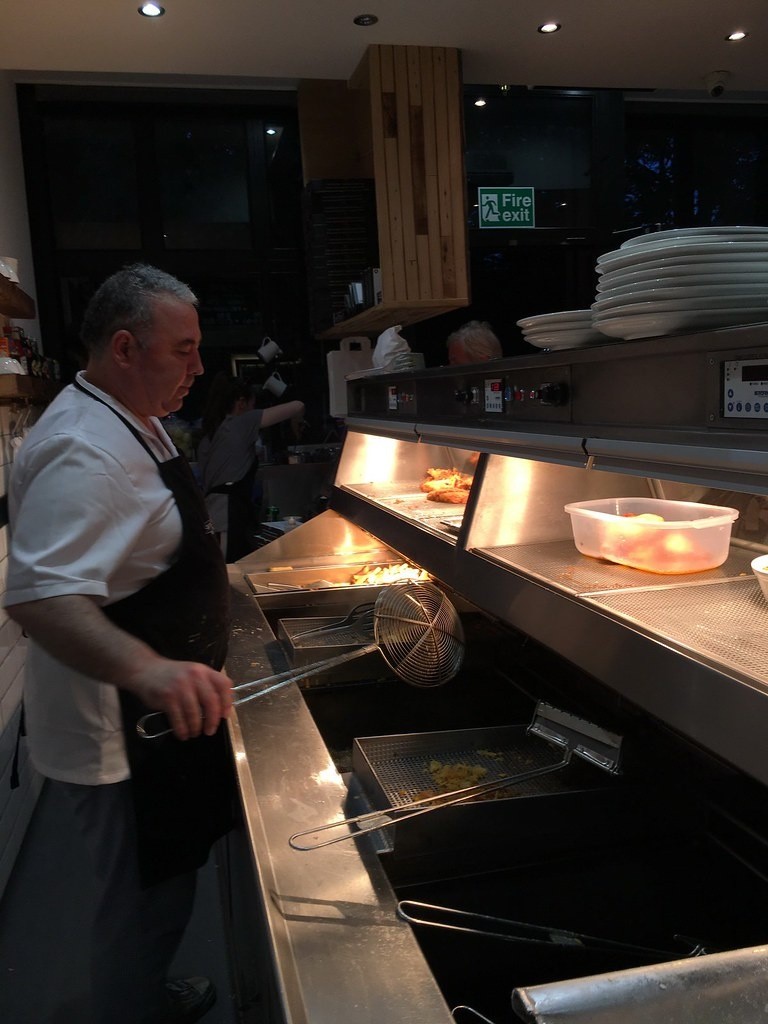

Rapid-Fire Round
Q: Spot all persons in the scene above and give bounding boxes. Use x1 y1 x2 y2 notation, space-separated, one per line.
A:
447 321 502 366
1 264 233 1024
175 359 305 565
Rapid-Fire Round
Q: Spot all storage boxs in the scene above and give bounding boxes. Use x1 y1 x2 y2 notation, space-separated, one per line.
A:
563 497 740 575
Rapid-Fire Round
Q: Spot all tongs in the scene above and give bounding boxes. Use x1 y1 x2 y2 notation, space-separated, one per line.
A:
291 602 394 642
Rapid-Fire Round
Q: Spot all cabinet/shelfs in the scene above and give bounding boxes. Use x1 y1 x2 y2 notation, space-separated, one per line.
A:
0 273 61 405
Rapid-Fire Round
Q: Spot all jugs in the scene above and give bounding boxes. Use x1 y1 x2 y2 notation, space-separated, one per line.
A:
263 372 286 397
258 337 283 363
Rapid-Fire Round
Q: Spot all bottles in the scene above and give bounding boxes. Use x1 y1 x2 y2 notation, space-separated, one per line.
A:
21 331 48 378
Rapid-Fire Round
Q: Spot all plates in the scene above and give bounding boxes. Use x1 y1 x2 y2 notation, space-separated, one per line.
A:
515 227 768 350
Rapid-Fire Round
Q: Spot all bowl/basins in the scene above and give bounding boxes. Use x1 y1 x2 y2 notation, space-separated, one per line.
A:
564 497 740 573
751 555 768 601
